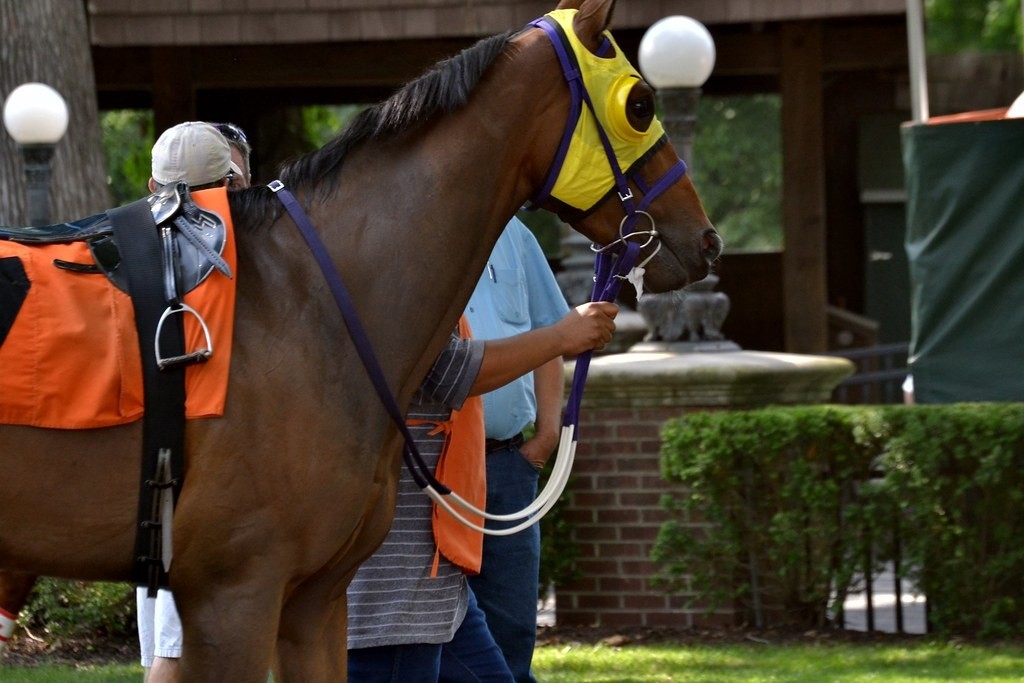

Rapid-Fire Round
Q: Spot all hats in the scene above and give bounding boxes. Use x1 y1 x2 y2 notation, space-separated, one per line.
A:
151 121 243 186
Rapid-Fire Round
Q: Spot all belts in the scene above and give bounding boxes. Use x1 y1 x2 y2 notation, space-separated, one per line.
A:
485 433 520 454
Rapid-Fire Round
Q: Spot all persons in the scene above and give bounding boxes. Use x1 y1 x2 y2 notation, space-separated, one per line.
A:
136 122 619 683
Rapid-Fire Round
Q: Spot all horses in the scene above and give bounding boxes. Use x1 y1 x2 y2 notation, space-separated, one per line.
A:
0 0 724 683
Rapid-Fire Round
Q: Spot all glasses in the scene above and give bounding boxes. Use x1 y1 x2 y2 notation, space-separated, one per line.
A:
215 125 246 142
